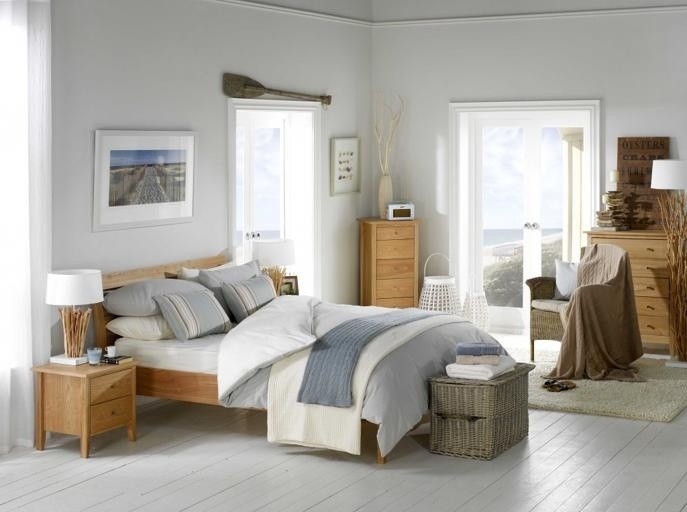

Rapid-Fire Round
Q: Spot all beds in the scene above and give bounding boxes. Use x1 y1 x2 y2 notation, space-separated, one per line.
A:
93 255 509 465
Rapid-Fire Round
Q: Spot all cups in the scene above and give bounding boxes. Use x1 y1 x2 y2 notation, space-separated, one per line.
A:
104 345 118 359
87 346 102 366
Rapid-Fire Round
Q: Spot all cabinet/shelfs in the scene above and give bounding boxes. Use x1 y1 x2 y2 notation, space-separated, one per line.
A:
359 220 421 307
583 230 676 357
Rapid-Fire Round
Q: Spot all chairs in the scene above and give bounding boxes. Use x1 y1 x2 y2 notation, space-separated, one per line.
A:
525 243 645 362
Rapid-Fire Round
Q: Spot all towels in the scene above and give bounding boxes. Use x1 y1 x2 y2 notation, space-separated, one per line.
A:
446 343 516 381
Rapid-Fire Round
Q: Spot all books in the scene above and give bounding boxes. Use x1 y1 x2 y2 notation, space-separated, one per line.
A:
98 352 134 366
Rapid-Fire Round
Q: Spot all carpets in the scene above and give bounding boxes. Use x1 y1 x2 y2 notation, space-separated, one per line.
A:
518 358 687 422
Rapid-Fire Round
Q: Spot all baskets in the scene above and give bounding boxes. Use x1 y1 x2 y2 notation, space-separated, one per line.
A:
428 362 536 460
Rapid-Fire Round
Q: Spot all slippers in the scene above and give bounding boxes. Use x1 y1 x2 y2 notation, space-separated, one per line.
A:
547 382 575 392
542 379 571 388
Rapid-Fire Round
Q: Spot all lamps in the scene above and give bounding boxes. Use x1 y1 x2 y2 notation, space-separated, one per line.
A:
45 269 105 367
651 159 687 190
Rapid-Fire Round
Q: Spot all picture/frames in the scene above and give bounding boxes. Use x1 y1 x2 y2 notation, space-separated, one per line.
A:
94 130 194 233
331 138 362 195
281 276 299 295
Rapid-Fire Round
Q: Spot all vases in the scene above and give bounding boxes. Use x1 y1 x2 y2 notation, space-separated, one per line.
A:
379 175 392 219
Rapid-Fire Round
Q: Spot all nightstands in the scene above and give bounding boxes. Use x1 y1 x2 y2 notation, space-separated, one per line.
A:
31 360 138 458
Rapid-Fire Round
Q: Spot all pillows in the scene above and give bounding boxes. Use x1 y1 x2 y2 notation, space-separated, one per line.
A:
552 261 577 300
103 259 277 341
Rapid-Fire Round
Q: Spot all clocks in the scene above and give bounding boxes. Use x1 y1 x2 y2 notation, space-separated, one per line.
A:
387 204 415 220
